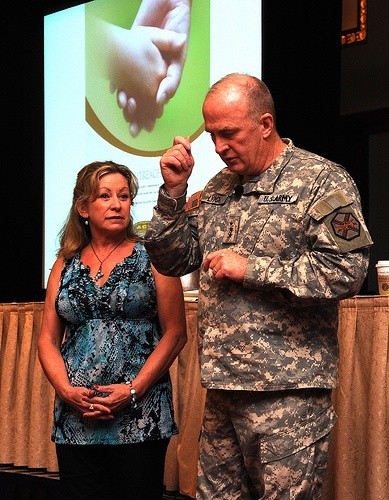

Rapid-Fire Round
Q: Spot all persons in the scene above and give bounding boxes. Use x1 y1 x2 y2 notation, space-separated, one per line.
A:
36 160 188 500
143 73 373 500
92 0 192 138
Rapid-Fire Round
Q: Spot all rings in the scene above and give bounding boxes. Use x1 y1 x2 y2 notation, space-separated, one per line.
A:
213 267 218 273
88 404 95 412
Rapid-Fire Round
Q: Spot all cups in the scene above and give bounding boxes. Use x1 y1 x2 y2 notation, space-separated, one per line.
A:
375 260 389 295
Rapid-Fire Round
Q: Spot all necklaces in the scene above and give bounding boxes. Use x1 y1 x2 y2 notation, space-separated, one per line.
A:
88 237 127 281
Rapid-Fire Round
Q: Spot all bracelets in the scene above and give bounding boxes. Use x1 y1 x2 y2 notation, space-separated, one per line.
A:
126 381 138 410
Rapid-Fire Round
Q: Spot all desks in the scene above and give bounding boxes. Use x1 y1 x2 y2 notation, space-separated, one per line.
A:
0 295 389 500
